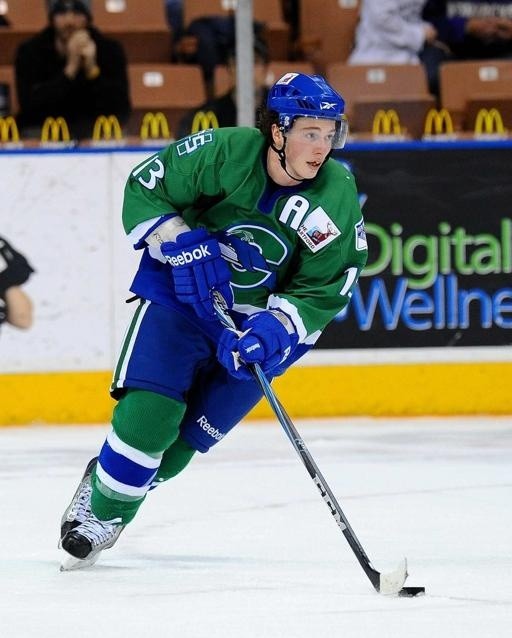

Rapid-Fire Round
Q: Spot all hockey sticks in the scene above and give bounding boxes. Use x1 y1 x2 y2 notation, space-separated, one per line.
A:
210 289 407 596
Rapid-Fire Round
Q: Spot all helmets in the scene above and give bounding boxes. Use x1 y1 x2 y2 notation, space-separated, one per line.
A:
258 71 348 149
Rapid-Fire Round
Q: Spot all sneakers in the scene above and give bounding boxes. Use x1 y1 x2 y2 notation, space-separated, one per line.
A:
60 457 125 558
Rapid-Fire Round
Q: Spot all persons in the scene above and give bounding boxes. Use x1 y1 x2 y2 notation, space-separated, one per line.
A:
57 69 370 557
14 0 133 140
174 36 271 142
346 0 437 66
0 237 38 332
419 0 512 99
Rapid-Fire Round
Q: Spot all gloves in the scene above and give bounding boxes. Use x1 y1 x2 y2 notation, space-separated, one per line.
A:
160 229 234 321
217 306 299 380
66 27 96 75
